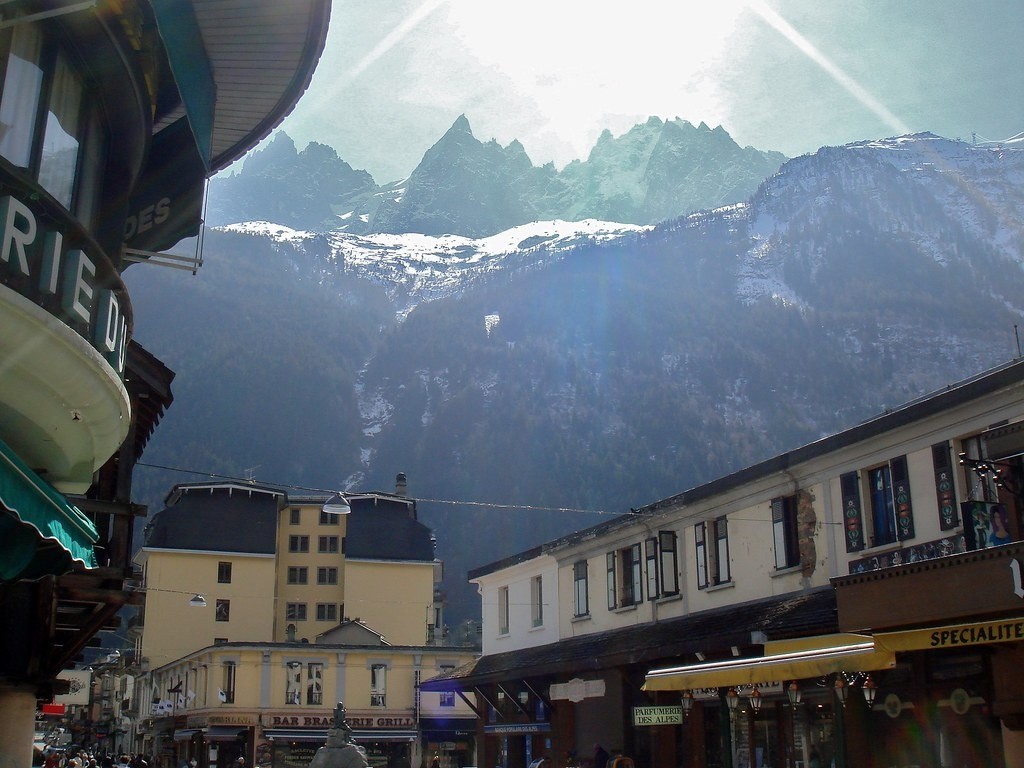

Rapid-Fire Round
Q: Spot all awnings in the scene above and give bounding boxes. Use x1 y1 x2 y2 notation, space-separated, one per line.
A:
421 718 477 737
174 730 201 741
644 643 895 690
204 728 242 741
0 440 99 584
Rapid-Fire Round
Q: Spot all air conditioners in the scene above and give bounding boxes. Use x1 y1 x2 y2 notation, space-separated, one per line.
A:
121 698 138 711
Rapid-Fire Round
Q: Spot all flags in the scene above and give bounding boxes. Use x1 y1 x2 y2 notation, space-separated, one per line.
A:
178 696 185 707
187 691 195 700
219 691 226 702
295 689 299 705
157 700 173 713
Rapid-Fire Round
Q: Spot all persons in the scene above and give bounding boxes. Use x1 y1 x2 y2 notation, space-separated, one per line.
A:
431 756 440 768
46 752 163 768
233 757 244 768
593 744 610 768
334 702 352 743
